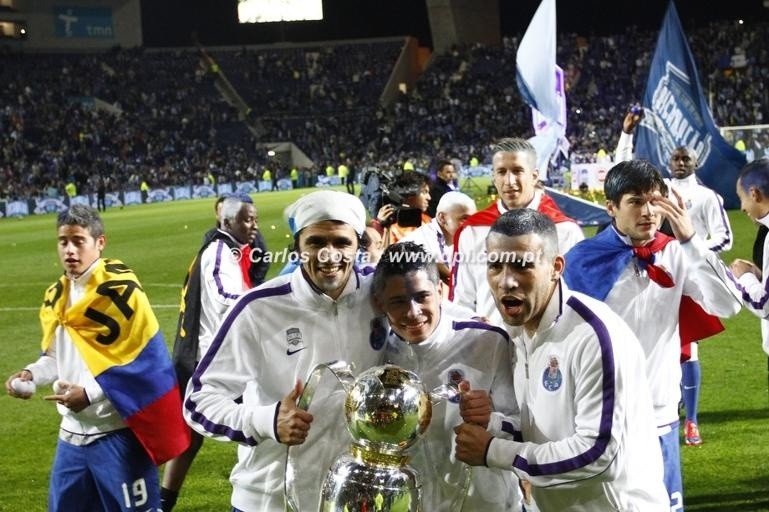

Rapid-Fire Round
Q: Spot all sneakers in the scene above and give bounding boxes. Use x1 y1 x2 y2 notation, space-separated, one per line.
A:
682 418 703 447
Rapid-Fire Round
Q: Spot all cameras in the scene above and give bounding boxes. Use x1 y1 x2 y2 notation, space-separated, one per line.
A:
627 105 645 119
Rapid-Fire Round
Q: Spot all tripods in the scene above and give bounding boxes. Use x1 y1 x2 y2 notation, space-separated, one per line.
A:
459 174 481 193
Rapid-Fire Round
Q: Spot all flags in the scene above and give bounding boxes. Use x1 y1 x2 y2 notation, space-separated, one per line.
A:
515 0 572 182
632 0 748 210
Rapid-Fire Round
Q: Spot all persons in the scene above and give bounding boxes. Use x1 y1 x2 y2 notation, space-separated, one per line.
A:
182 189 492 512
5 203 192 512
454 208 672 512
371 170 433 250
159 191 259 512
357 34 533 170
2 39 356 212
395 191 476 302
203 193 270 288
730 158 769 394
556 27 660 165
697 86 769 165
562 160 741 512
372 242 540 511
424 160 454 218
614 102 734 446
679 16 769 87
448 137 585 334
